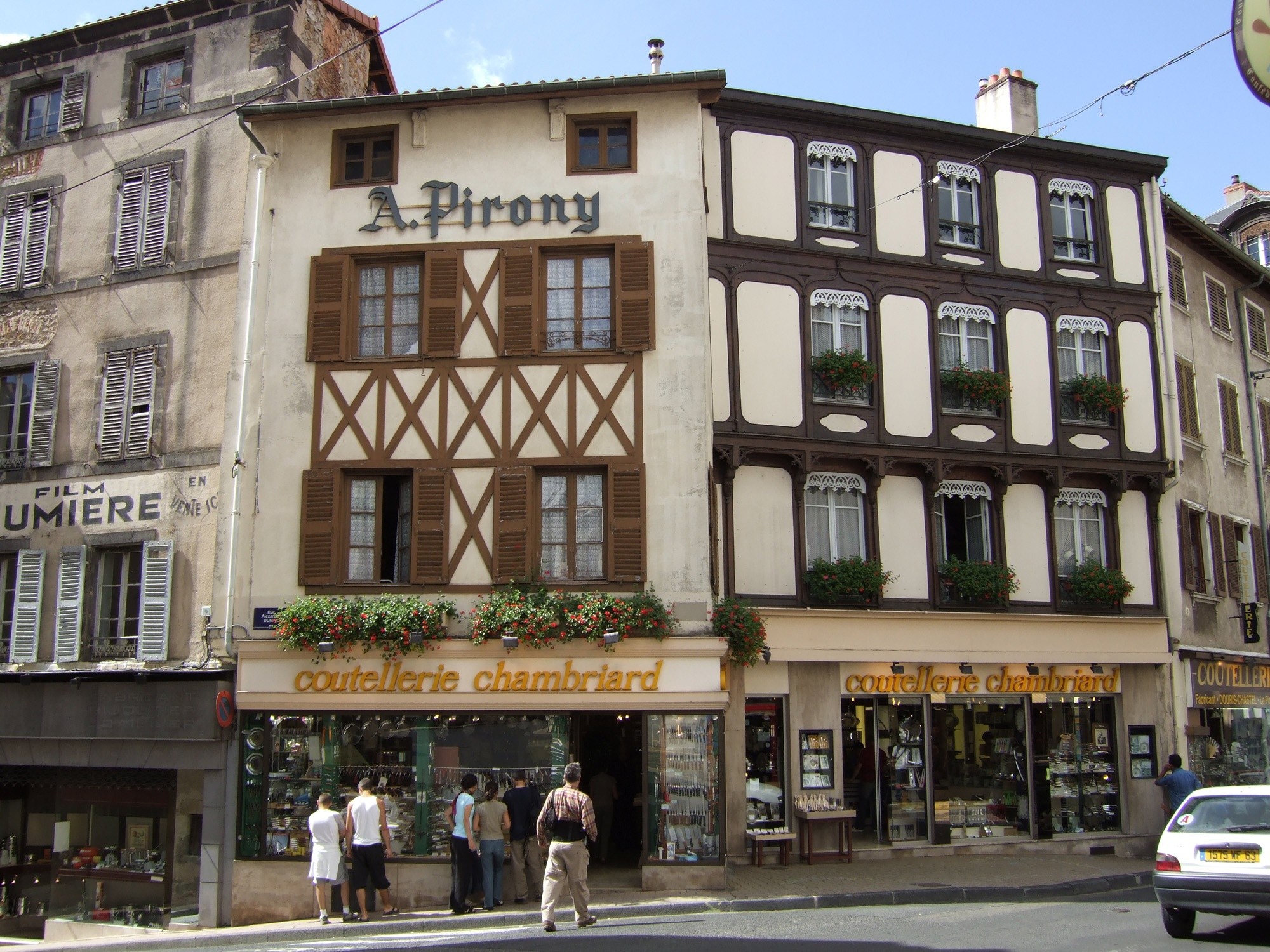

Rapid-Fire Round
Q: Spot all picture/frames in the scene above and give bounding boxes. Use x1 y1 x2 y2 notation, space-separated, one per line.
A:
667 842 675 860
128 824 148 850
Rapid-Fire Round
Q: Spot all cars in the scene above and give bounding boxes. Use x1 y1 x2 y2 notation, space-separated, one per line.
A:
1151 784 1270 938
666 768 783 827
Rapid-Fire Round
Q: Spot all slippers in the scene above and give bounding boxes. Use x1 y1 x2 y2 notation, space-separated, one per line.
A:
358 915 369 922
383 907 399 915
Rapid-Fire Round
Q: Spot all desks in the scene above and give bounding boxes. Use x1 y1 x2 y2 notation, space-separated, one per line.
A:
751 839 790 867
799 817 853 866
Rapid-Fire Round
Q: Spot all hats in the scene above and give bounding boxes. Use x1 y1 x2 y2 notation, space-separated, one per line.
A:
461 774 477 790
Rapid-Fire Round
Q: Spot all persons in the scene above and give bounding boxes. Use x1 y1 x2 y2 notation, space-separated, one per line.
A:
608 744 640 849
309 793 359 925
344 778 399 922
340 785 399 858
536 762 597 932
931 730 1041 800
444 774 478 914
844 735 913 834
585 768 618 864
472 781 510 910
1155 754 1203 814
502 770 542 904
756 747 770 772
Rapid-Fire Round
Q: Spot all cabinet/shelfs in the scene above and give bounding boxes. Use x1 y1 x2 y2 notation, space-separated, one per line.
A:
891 743 924 790
799 729 836 790
0 862 52 933
1048 749 1118 833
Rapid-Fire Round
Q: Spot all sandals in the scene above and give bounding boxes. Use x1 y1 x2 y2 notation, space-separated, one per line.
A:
454 905 476 914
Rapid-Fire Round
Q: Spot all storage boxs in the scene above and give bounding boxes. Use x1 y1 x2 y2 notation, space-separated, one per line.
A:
746 833 796 840
795 808 856 820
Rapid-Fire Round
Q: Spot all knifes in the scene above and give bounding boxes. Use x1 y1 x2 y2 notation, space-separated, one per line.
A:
665 725 706 849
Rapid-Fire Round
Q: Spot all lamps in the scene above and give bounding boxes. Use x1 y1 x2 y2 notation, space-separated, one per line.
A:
20 673 153 686
967 697 1091 709
1026 663 1039 675
409 628 423 644
503 632 518 648
318 637 334 652
604 628 620 644
1089 663 1103 674
760 645 770 664
890 662 904 674
959 662 973 674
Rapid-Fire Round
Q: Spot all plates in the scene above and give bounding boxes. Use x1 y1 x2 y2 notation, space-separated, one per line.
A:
245 753 263 775
342 718 410 744
246 728 264 749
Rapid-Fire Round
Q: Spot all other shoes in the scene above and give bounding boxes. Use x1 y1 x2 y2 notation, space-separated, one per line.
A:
536 894 542 902
514 898 527 905
493 899 504 907
483 906 494 910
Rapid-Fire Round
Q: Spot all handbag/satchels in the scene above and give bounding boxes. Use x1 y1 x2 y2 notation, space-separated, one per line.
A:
544 788 557 832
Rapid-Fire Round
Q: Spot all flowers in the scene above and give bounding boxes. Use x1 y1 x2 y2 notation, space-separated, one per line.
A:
799 348 1135 604
267 578 768 666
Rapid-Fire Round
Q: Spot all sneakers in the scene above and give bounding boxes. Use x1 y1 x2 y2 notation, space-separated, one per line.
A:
343 910 359 922
543 920 556 932
577 916 597 928
320 915 328 925
